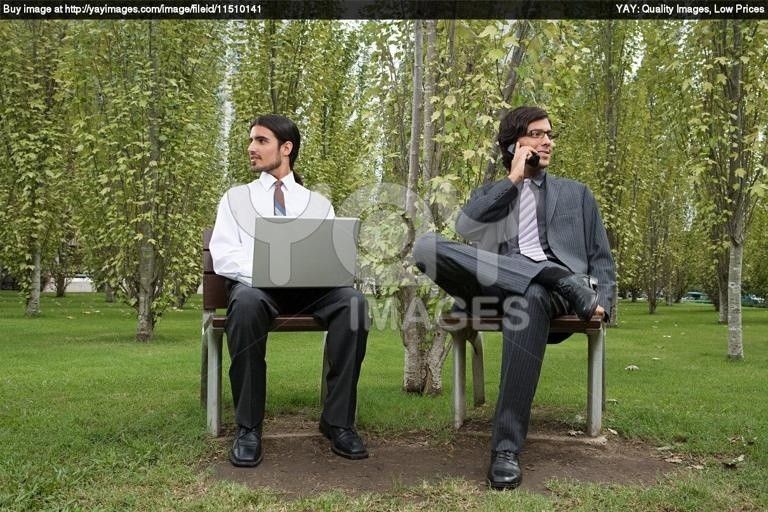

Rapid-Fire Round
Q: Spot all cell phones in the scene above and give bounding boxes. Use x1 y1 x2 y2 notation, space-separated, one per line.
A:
507 144 540 168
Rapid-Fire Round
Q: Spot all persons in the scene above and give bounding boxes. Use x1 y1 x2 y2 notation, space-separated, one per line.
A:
210 115 369 468
413 107 617 490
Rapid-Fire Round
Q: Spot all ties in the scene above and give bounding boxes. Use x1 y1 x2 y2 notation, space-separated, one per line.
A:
518 179 548 262
274 181 286 217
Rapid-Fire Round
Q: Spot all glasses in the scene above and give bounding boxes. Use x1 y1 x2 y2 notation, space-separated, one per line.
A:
527 129 559 139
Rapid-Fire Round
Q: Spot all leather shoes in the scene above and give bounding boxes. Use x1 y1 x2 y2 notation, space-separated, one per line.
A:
320 416 369 459
555 274 598 321
487 450 521 487
229 424 263 466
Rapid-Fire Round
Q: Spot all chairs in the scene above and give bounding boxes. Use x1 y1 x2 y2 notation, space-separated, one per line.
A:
437 227 608 438
199 228 374 439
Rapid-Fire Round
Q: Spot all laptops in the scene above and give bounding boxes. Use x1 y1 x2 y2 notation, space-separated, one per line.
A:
251 217 360 288
